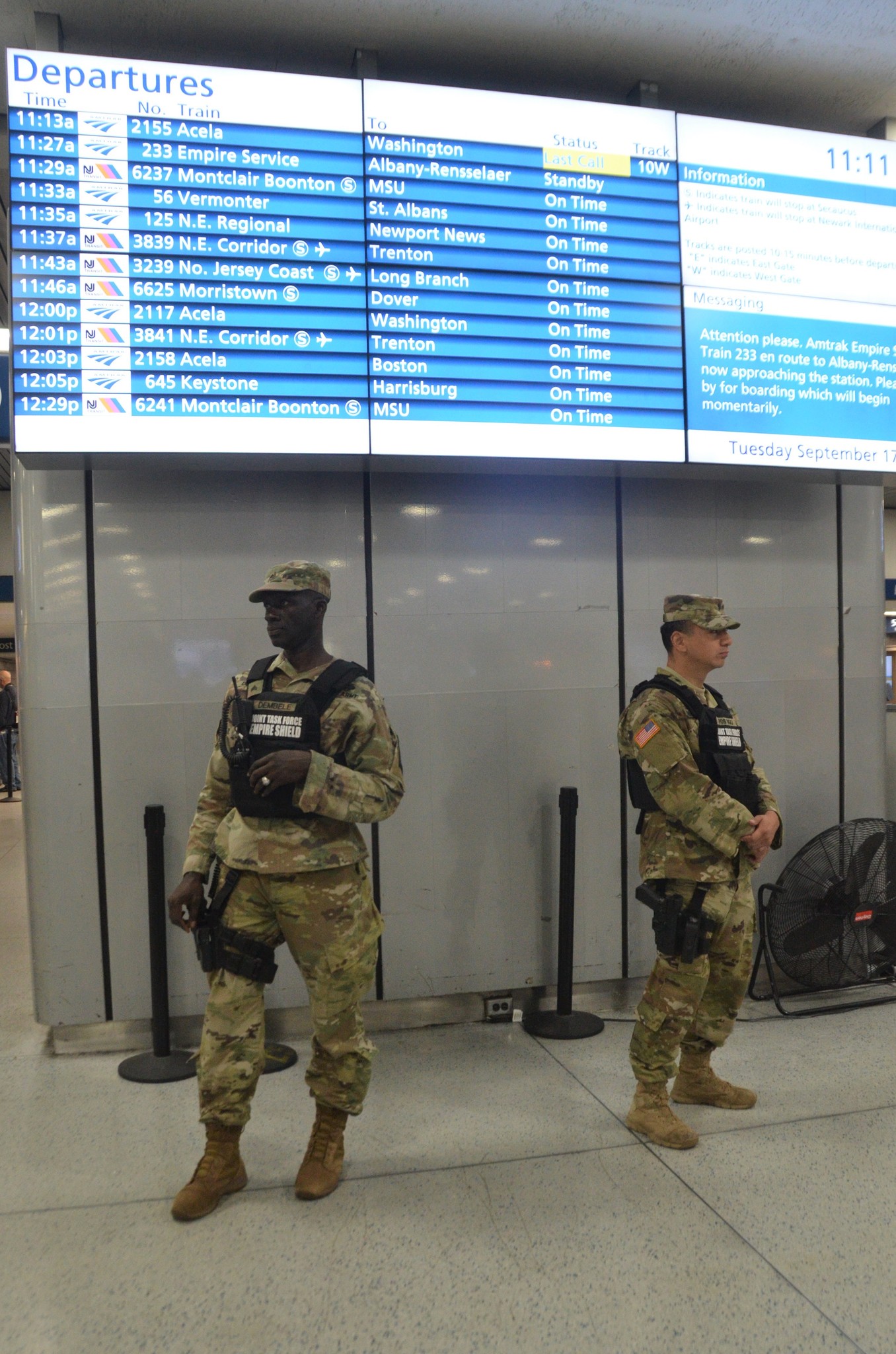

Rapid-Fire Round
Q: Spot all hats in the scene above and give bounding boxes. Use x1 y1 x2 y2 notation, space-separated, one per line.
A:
248 560 331 603
663 594 740 631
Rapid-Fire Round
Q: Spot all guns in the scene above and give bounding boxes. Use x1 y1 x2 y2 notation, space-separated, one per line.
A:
186 899 212 973
634 884 683 958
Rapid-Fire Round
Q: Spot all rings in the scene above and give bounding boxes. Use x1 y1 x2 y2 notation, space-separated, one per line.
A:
261 775 270 786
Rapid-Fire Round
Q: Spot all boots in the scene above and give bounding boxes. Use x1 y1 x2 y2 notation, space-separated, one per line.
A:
670 1048 756 1109
170 1122 247 1220
293 1103 348 1200
625 1081 699 1150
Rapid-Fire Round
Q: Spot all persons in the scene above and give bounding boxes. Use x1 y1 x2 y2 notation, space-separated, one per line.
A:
618 592 782 1149
168 561 403 1220
0 670 21 791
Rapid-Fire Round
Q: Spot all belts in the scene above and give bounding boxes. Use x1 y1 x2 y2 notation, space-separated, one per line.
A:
0 729 13 734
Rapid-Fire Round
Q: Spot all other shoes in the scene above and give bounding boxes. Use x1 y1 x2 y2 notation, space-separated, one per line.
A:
0 786 17 792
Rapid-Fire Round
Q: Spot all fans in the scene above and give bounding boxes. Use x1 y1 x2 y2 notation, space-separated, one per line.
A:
749 817 896 1017
